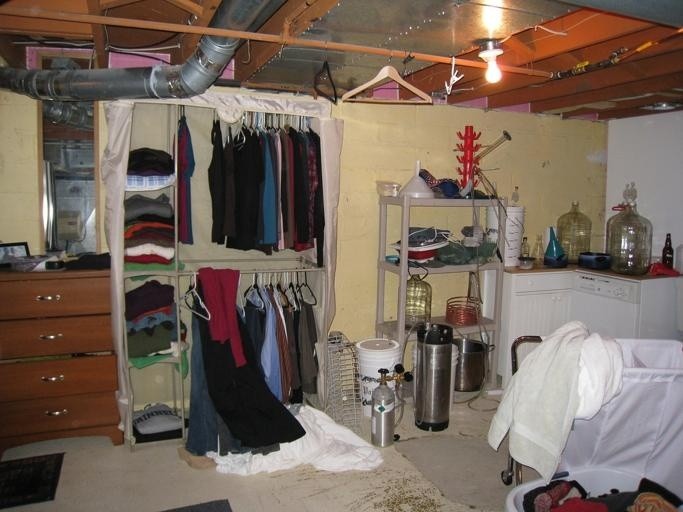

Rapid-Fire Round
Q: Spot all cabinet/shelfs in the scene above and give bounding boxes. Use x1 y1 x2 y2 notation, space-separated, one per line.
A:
377 193 507 400
0 269 124 458
483 268 573 388
102 90 344 452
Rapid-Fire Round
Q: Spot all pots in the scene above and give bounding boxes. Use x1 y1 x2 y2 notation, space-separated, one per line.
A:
577 251 612 270
452 338 496 394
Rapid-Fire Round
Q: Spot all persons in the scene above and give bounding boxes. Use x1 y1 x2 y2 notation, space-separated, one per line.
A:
10 246 19 259
1 248 13 261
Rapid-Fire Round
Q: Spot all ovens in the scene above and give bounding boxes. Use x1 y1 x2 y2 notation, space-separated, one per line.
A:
574 265 682 341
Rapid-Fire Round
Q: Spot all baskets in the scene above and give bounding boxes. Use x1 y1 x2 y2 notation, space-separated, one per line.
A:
324 333 363 427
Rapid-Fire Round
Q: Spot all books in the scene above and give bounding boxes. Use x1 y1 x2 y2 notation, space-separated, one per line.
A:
10 255 59 272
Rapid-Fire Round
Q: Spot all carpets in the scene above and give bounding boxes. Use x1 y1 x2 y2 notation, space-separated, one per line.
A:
0 452 64 509
159 498 233 512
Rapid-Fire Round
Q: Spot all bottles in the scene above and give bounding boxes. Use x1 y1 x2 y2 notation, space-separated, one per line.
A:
511 186 520 207
663 233 673 267
531 232 545 270
521 237 529 258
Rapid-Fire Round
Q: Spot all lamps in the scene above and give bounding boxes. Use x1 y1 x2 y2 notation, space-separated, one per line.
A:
478 41 504 84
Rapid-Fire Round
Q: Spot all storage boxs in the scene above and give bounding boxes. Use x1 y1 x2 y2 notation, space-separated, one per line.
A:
506 465 683 512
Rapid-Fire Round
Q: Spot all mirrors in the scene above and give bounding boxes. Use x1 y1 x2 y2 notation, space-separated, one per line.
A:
37 49 99 255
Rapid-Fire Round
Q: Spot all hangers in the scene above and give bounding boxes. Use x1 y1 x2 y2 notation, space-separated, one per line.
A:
210 111 317 149
341 49 433 105
314 40 337 103
180 267 317 321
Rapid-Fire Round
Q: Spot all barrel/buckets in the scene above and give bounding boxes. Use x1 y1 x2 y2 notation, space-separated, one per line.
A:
413 347 458 411
357 339 399 416
558 200 592 264
604 203 655 277
487 205 525 268
